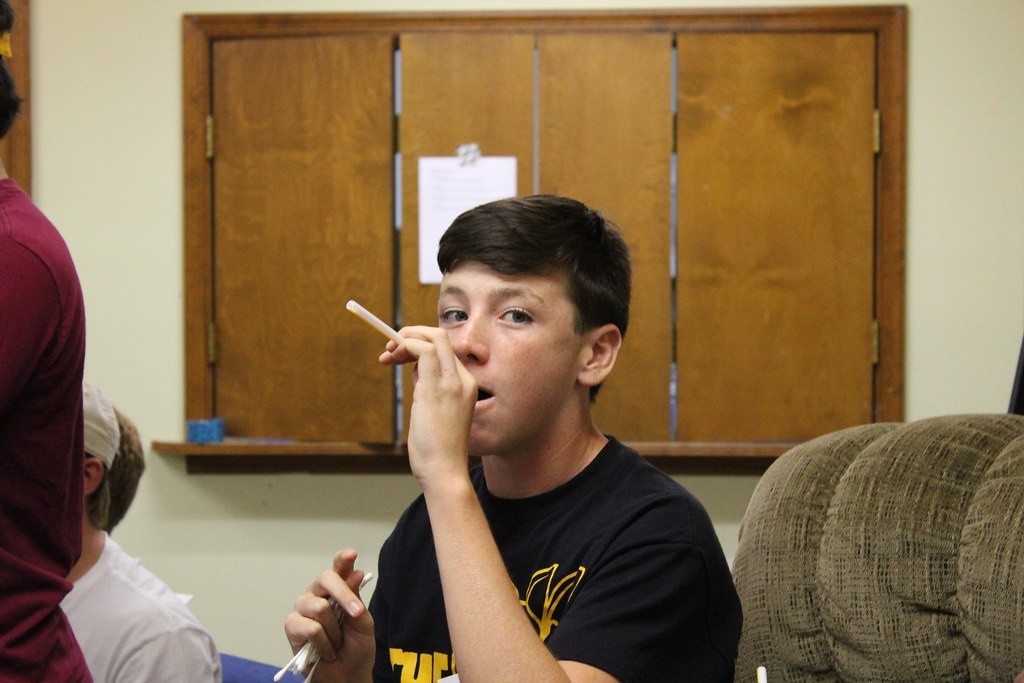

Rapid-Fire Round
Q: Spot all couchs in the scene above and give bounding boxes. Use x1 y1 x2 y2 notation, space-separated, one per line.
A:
729 414 1024 683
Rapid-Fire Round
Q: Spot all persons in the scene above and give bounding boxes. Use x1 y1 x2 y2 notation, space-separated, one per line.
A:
60 380 223 683
0 1 93 683
286 193 742 683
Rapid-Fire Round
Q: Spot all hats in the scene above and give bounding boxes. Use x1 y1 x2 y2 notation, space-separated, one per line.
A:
81 374 121 470
